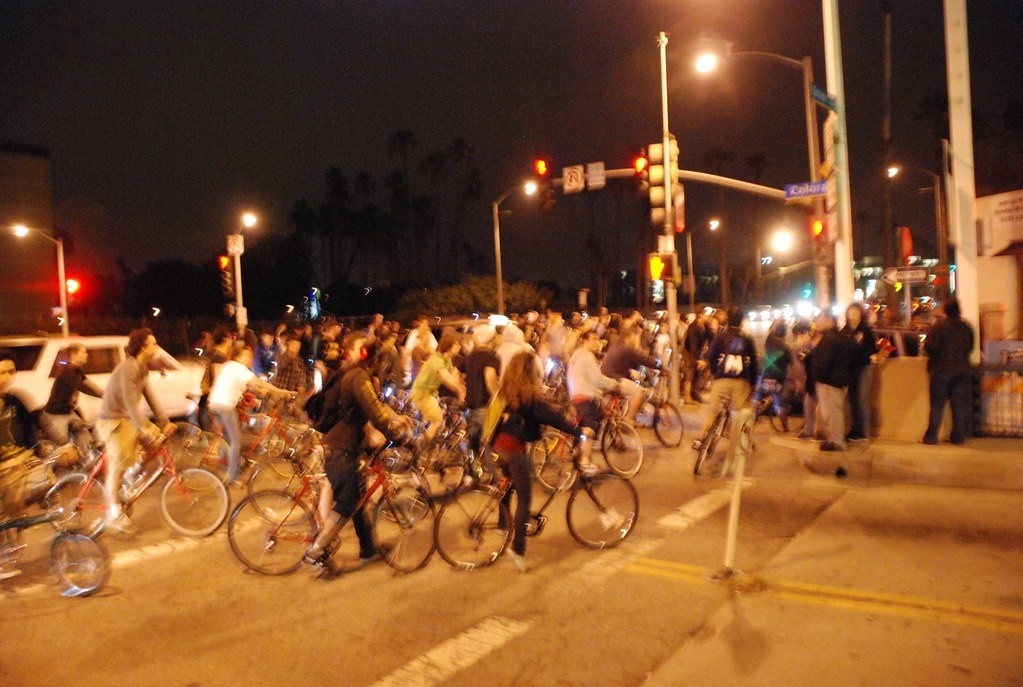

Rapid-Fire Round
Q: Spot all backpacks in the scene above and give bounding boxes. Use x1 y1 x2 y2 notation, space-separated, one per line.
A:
301 364 363 434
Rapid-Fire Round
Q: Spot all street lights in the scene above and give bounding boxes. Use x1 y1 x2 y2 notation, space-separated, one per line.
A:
13 225 69 335
686 217 721 312
694 48 832 311
234 213 257 338
888 164 951 302
493 179 539 315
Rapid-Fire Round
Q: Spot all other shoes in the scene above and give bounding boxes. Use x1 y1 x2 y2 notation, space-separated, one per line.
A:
361 543 393 563
792 431 816 440
506 548 526 573
809 434 828 443
816 443 847 454
944 436 966 444
301 548 334 579
622 416 638 427
104 511 139 534
917 437 939 444
847 435 872 441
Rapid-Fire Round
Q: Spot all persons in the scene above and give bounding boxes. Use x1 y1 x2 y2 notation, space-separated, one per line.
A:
0 259 882 579
922 296 974 446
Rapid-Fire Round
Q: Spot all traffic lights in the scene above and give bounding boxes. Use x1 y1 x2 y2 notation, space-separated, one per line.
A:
224 304 233 316
649 143 665 226
634 158 649 194
535 159 548 176
648 256 675 279
220 257 233 295
812 219 827 261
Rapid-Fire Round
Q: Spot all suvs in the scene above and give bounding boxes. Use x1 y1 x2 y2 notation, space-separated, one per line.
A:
0 334 212 429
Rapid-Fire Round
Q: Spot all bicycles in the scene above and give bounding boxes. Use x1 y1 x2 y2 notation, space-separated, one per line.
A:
0 371 687 598
690 390 807 475
